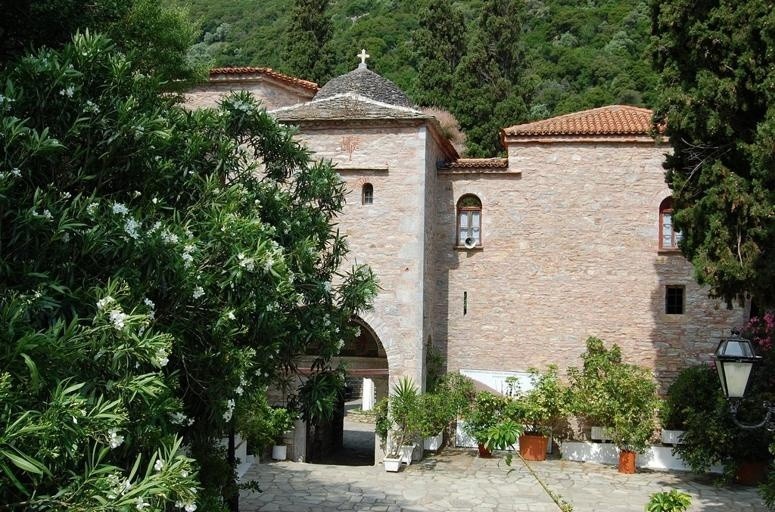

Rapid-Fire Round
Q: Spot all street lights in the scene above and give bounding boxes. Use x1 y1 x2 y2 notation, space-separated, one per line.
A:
711 326 775 434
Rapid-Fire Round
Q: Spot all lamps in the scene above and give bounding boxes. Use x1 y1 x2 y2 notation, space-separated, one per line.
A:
709 328 761 400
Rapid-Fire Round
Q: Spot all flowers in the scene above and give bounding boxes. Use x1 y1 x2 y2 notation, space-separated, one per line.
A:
710 314 775 370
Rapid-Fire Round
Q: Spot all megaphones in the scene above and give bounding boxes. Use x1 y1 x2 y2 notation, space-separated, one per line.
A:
464 236 476 249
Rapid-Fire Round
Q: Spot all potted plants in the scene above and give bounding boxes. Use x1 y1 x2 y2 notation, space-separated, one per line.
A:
569 359 661 474
656 363 769 487
457 389 517 455
373 373 477 473
504 365 569 462
266 408 296 460
